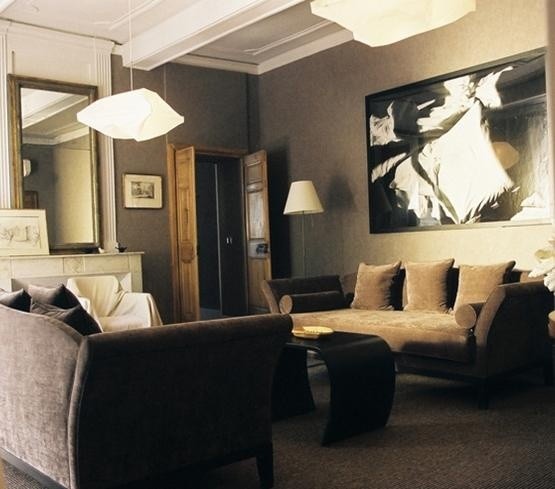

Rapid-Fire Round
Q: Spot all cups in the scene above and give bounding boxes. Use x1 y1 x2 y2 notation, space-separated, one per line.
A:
115 241 128 252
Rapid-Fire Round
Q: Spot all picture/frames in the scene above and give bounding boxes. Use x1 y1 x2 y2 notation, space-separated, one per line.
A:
0 208 50 257
363 46 549 235
23 190 39 209
122 172 163 210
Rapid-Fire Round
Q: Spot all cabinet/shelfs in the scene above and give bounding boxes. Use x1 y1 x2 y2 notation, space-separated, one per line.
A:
0 250 145 295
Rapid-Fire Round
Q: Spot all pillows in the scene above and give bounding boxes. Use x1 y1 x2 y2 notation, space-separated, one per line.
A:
278 291 342 314
28 283 79 308
453 260 516 312
402 258 454 316
349 262 401 310
455 302 485 330
0 287 30 311
30 296 102 335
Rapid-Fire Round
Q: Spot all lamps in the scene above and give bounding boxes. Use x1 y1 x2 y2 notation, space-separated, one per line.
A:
311 0 477 49
74 0 184 142
284 180 324 279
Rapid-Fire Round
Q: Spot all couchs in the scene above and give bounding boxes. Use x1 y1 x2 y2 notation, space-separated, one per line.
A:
0 305 294 489
259 272 552 400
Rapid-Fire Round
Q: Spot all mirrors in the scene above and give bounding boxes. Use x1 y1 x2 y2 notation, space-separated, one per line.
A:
7 73 103 254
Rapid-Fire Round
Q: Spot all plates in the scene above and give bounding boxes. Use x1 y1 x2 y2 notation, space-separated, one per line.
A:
293 326 334 339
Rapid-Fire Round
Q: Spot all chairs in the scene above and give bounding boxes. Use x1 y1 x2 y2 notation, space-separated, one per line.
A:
66 275 163 333
